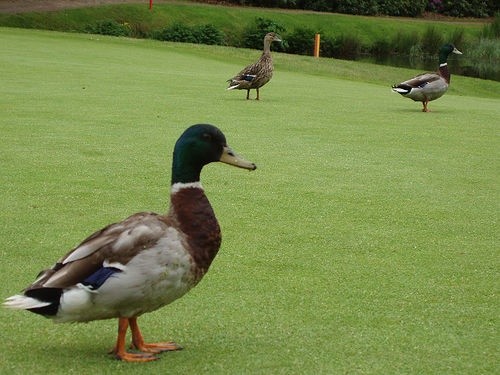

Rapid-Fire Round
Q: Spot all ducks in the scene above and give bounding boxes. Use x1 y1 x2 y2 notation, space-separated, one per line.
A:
391 42 463 112
2 123 257 363
225 32 282 101
